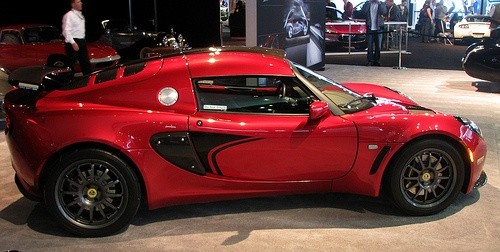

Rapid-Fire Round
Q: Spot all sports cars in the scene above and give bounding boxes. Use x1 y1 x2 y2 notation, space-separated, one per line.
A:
3 46 488 239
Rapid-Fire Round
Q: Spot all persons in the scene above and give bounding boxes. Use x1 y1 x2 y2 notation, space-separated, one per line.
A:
326 0 337 20
364 0 388 66
62 0 93 74
488 4 500 37
342 0 353 18
420 0 447 43
382 0 409 48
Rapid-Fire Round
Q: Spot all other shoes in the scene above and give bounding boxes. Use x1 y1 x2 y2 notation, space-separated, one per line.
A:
423 41 430 43
366 63 374 66
375 63 382 67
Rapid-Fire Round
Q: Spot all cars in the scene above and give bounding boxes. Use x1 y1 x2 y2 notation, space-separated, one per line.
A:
462 28 500 82
0 22 123 85
93 18 166 52
454 14 500 41
324 6 368 51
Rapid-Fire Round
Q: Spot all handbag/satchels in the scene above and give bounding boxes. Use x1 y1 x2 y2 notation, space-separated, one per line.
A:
419 12 429 23
